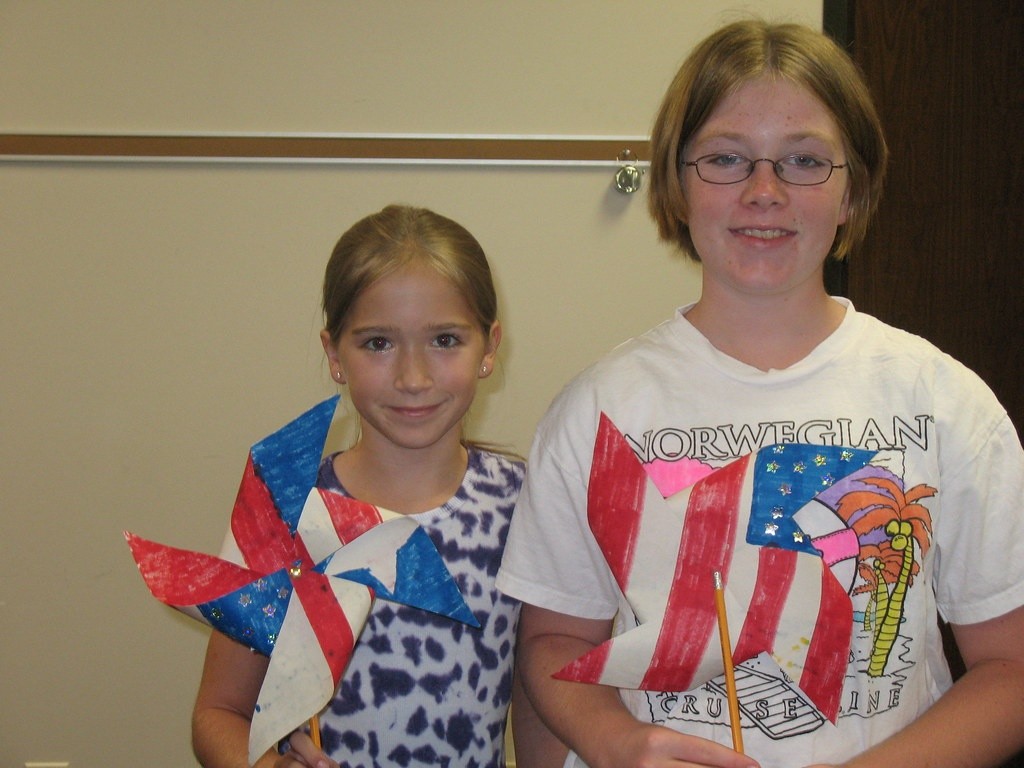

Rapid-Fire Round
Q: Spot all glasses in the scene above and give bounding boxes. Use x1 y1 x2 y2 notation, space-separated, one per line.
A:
680 153 848 186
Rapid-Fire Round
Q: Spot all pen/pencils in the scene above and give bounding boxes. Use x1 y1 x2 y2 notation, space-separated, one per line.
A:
310 715 321 748
713 571 745 752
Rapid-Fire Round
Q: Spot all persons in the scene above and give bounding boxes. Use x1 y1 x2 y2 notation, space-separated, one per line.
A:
494 20 1024 768
190 205 527 768
